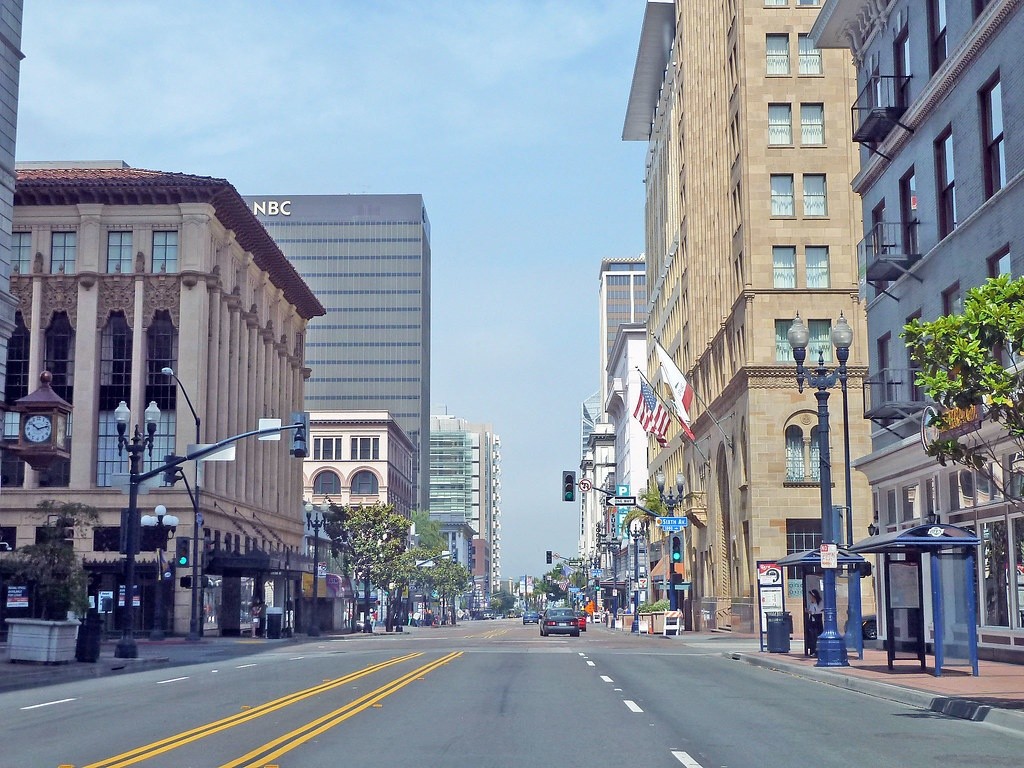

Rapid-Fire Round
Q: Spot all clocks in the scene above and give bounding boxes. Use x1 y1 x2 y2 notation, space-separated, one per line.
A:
24 415 52 442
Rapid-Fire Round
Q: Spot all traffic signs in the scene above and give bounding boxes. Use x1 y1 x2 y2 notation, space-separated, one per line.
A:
604 496 639 507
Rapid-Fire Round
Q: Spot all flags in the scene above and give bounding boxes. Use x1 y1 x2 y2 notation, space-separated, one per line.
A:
558 582 567 591
633 376 672 450
655 340 698 443
562 563 574 576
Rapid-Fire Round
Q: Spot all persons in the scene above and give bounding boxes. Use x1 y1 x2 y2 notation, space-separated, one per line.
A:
344 609 353 628
249 597 260 638
408 609 441 626
370 608 378 630
805 588 823 657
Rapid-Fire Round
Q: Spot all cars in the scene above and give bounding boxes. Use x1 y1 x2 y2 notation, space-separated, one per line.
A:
538 607 580 636
576 611 587 631
522 611 539 625
474 609 519 620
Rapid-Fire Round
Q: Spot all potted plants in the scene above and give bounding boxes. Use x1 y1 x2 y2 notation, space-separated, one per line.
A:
0 499 103 666
650 599 670 633
636 599 652 632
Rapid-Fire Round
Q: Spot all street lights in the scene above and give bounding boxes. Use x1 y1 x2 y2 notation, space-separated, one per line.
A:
655 471 686 633
618 523 646 633
138 503 179 644
832 311 868 658
113 398 160 661
588 551 601 611
162 365 204 642
788 310 852 667
303 496 328 636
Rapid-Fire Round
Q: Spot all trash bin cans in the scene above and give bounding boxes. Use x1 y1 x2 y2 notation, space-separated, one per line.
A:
267 613 283 638
765 611 791 652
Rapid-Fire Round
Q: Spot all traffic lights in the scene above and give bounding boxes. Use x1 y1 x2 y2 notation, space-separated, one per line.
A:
180 576 191 587
546 550 552 565
670 533 684 565
595 577 602 593
561 470 577 501
164 452 187 486
178 555 191 570
